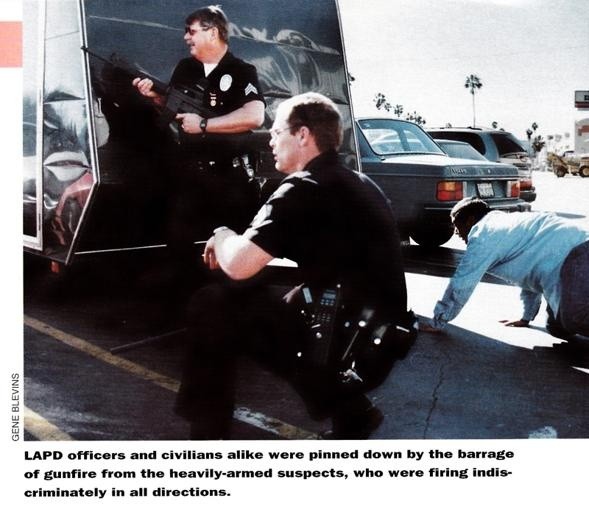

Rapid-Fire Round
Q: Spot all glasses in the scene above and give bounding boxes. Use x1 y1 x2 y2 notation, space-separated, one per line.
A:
269 123 306 139
185 26 215 35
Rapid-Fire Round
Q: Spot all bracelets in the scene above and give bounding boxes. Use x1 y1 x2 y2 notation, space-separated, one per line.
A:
212 226 229 234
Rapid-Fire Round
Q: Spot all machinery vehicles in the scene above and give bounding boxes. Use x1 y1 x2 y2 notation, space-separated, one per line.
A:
548 150 589 177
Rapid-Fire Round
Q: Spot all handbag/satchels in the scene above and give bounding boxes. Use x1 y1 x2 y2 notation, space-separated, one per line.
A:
376 312 419 360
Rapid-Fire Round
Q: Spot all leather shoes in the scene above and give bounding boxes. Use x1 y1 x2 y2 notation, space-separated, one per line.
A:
317 405 385 439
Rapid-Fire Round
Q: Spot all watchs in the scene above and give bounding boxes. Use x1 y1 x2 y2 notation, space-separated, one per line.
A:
198 119 209 133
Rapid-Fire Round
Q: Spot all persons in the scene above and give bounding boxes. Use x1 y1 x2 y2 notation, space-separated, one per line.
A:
169 91 410 438
414 195 588 358
129 4 266 313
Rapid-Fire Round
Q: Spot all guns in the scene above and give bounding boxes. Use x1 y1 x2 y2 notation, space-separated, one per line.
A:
109 329 176 353
79 45 254 147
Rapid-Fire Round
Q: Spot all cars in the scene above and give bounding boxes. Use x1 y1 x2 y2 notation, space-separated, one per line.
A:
355 115 536 246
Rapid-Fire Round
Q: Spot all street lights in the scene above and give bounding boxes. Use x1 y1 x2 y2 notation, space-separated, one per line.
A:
464 75 482 128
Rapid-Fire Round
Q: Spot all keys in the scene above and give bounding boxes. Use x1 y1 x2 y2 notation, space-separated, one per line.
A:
338 362 365 386
